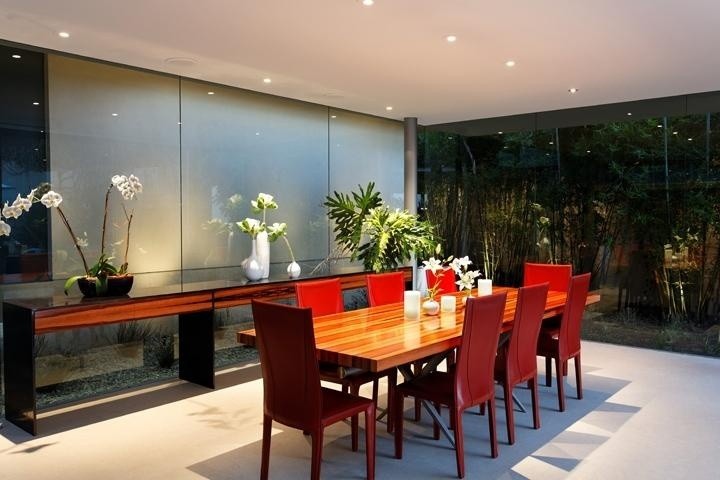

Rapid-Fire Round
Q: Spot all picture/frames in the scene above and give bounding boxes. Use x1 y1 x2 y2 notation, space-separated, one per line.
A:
203 246 227 271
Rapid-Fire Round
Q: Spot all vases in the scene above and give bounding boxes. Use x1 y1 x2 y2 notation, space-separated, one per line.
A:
239 229 270 283
76 272 133 293
462 294 476 306
421 299 439 316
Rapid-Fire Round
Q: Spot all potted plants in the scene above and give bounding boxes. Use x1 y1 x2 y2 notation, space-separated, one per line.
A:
324 182 435 270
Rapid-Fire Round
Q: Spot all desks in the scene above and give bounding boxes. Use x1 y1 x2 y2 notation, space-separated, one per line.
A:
235 284 600 459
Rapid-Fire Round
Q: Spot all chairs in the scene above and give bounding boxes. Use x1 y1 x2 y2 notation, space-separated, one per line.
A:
251 299 375 480
523 262 573 292
366 270 455 423
536 272 592 413
295 278 395 452
394 291 507 479
448 282 549 445
427 269 456 296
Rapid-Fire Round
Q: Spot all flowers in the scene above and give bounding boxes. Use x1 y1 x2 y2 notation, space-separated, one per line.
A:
418 254 472 300
0 173 143 272
453 269 482 292
237 190 305 276
202 190 241 245
